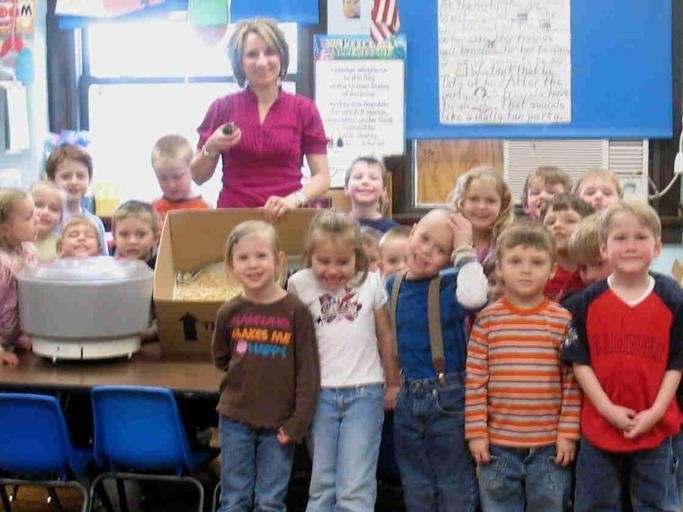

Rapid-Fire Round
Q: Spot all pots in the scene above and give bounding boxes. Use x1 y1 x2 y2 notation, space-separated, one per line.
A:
17 256 155 366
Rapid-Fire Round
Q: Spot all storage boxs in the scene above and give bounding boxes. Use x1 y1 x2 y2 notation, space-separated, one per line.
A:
153 207 330 363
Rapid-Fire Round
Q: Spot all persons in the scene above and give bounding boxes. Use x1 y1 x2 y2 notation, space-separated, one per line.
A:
565 199 683 511
213 223 318 512
568 216 610 283
539 197 596 298
288 207 400 512
192 17 331 218
360 222 382 269
384 208 490 512
46 142 108 256
382 224 414 275
57 220 102 259
522 165 570 222
465 219 581 511
343 0 361 20
573 169 620 209
110 200 156 271
451 165 512 266
30 180 65 265
153 135 211 220
333 156 401 233
0 189 39 365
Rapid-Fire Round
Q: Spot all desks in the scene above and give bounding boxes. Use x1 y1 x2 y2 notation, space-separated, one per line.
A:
1 351 226 394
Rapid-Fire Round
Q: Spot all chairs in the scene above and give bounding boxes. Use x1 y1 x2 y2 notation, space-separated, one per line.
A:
0 393 114 512
87 386 220 512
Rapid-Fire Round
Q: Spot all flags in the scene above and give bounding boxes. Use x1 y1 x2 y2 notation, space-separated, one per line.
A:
370 1 400 46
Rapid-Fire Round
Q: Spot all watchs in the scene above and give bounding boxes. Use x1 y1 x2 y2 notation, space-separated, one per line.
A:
293 188 311 206
